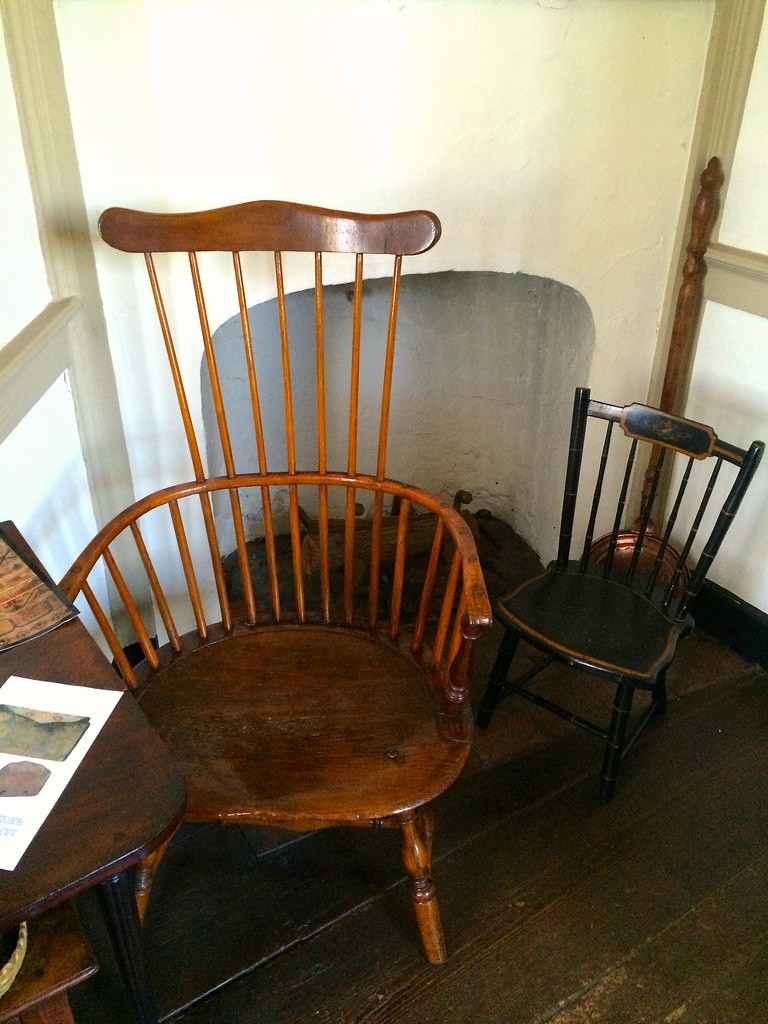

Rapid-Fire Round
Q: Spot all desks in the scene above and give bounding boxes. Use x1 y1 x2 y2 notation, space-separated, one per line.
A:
0 519 189 1022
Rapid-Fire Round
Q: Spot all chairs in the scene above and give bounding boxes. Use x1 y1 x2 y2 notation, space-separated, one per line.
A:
55 197 491 966
470 388 764 812
